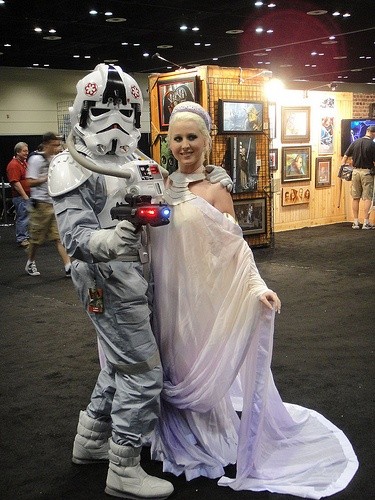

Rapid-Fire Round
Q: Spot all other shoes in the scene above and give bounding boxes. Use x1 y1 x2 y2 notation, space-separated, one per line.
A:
362 223 375 230
352 224 359 229
19 238 30 247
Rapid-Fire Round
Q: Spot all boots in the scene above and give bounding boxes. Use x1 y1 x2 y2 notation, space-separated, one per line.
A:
105 437 175 500
72 410 113 464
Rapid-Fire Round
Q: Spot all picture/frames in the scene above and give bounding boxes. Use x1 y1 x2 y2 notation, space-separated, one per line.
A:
159 134 178 175
157 75 197 131
232 197 267 237
267 103 277 139
269 149 278 170
316 157 332 187
281 106 310 143
218 99 264 135
281 145 311 183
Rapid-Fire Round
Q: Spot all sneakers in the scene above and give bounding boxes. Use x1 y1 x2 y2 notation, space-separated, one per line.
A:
65 268 71 277
25 259 40 276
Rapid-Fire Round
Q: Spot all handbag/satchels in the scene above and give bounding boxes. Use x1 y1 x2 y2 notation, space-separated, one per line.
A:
337 164 351 181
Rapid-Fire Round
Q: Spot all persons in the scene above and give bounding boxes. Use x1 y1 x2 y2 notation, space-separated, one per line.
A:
25 133 72 277
7 142 30 245
340 125 375 230
48 63 235 500
149 101 359 500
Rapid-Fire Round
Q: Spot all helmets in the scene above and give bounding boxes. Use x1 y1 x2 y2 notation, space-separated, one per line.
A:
68 63 143 157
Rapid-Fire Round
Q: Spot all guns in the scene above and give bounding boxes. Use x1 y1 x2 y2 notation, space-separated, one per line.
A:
109 193 171 233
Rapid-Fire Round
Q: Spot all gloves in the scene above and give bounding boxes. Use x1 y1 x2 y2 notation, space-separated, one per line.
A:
89 220 136 262
206 165 232 192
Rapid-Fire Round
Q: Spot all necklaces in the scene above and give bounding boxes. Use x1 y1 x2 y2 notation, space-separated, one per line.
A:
366 136 372 138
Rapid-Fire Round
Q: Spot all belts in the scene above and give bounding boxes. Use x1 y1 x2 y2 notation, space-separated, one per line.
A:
353 167 370 169
